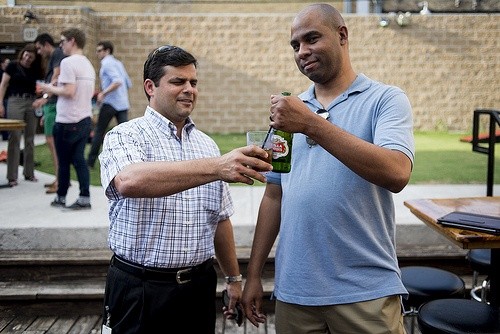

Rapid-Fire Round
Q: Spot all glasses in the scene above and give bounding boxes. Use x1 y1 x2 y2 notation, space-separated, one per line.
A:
60 39 69 43
146 45 180 76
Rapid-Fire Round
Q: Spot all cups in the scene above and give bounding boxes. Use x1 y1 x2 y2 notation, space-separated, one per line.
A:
35 106 42 117
36 79 44 91
246 131 274 172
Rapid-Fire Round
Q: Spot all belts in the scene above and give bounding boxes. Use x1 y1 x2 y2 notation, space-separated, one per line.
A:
112 255 214 285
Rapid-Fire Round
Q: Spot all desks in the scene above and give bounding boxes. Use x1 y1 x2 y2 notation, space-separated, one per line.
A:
403 197 500 301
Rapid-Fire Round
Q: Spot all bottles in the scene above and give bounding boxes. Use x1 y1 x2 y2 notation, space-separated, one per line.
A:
270 92 293 173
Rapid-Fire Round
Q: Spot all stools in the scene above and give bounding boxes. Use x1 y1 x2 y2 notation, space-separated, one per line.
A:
398 248 500 334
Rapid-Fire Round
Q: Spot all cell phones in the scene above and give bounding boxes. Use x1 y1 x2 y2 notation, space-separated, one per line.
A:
222 290 243 327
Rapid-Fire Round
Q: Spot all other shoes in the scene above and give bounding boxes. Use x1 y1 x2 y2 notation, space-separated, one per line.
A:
8 180 18 187
65 196 91 210
50 195 66 207
44 181 58 193
25 175 38 181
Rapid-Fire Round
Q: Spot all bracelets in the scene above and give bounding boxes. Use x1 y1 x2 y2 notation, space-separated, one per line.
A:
225 274 242 284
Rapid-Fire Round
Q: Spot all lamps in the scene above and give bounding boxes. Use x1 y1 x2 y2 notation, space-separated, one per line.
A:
23 10 35 24
417 1 430 15
380 11 401 27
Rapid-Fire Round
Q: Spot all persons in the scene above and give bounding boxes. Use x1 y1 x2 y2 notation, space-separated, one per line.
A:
240 3 415 334
98 44 273 334
0 28 131 211
